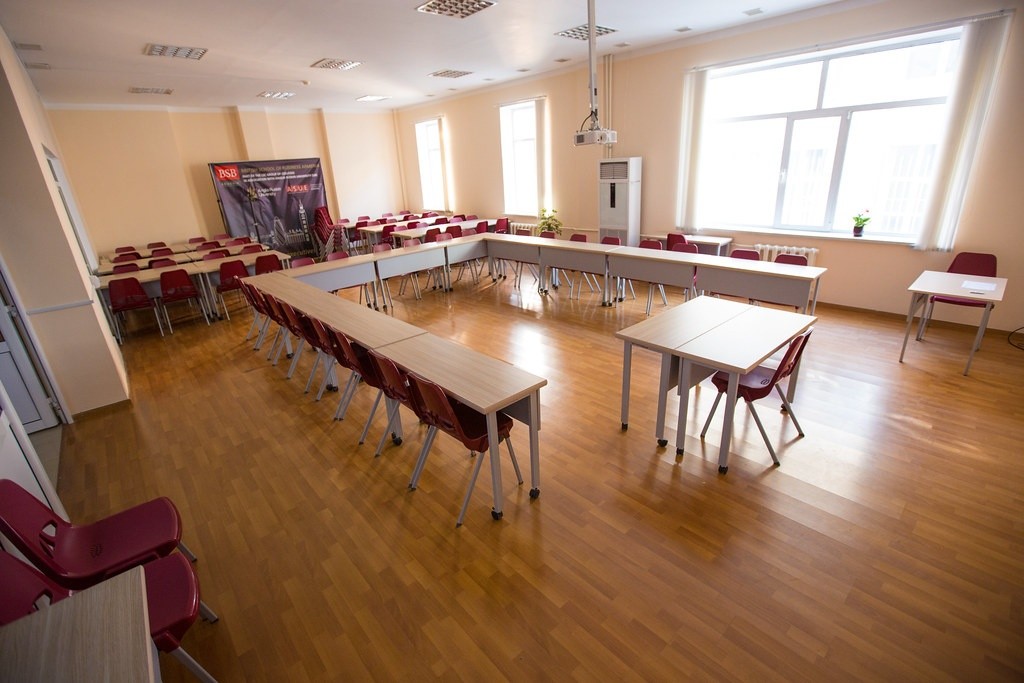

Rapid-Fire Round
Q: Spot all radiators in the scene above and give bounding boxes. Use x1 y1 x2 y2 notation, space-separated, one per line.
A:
730 243 819 266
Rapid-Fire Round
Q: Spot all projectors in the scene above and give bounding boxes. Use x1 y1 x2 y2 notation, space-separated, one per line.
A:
574 130 617 146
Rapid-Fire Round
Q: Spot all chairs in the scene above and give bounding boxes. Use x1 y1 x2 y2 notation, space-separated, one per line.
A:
0 210 804 683
919 252 997 352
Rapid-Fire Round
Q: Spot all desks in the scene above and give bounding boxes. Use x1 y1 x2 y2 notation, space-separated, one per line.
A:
0 565 163 683
97 213 1009 520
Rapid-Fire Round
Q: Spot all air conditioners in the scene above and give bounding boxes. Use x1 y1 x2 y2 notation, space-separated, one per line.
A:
598 157 642 247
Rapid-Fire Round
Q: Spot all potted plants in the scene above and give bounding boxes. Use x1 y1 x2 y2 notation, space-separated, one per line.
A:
853 210 870 237
